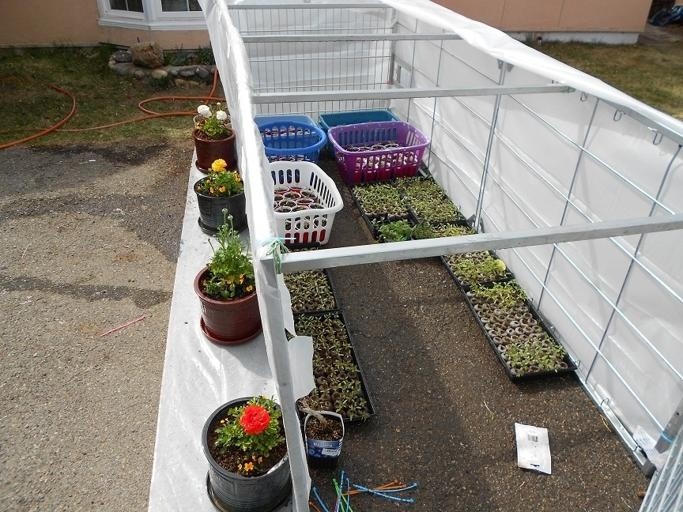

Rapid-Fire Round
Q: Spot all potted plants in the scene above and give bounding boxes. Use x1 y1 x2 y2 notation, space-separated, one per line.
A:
192 207 269 345
283 244 379 463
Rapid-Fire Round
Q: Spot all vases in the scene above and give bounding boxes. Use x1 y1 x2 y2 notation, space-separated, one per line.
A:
194 178 248 236
191 130 237 173
202 398 292 507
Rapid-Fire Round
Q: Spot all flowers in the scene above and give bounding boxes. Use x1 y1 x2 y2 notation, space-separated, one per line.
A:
193 105 230 139
216 398 286 469
201 156 240 195
